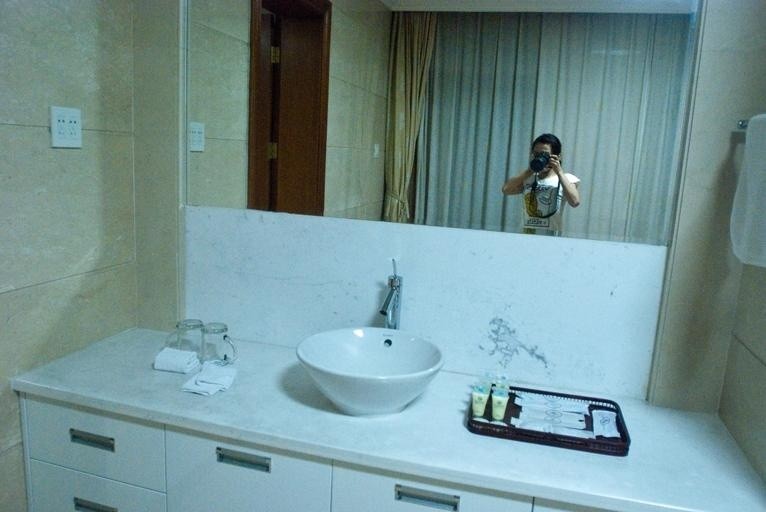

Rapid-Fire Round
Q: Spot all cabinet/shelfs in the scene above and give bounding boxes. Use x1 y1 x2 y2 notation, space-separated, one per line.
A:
8 378 167 512
332 449 535 510
166 411 333 511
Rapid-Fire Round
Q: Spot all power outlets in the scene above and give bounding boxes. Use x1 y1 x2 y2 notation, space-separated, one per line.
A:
49 106 83 148
187 120 204 152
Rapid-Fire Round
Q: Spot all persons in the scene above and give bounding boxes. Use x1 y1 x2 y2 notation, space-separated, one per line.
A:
502 133 581 237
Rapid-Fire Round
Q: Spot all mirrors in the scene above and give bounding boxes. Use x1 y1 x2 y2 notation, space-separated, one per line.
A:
184 1 704 247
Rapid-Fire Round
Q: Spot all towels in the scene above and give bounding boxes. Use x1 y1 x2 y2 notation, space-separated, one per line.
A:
725 113 766 269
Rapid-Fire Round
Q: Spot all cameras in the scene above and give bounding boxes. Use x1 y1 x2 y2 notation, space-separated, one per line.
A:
530 151 551 172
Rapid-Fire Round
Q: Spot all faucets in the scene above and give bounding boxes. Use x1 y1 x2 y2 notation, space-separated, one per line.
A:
379 258 402 330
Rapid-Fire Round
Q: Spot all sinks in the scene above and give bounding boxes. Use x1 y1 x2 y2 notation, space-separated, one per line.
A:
297 327 442 417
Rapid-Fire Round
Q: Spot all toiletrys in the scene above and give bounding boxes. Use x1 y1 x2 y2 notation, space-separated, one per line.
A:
472 372 510 420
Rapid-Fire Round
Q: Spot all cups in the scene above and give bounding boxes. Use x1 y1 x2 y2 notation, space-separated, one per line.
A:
166 319 236 368
